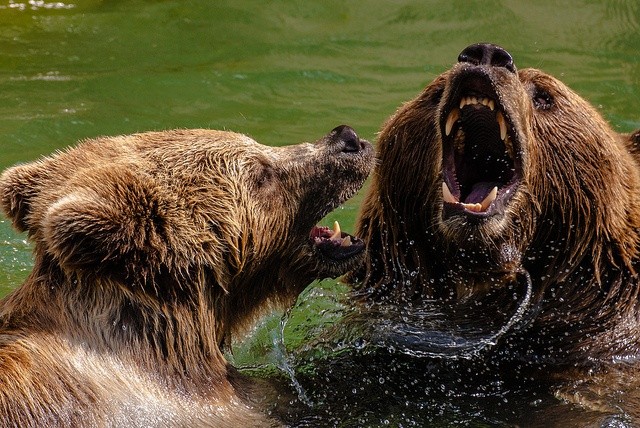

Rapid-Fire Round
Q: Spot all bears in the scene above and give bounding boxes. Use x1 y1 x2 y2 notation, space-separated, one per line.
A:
0 124 384 427
337 41 639 427
